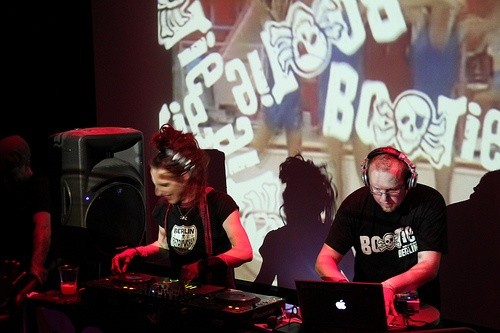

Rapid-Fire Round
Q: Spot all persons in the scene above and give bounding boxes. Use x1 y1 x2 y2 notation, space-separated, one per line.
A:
314 145 448 319
0 134 52 284
111 124 253 290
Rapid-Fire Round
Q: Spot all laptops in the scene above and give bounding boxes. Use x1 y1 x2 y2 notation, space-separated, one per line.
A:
295 280 407 332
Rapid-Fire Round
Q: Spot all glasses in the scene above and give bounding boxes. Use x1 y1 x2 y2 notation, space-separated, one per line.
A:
368 180 407 197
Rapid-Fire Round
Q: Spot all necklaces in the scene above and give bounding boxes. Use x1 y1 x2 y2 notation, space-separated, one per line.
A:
177 203 196 221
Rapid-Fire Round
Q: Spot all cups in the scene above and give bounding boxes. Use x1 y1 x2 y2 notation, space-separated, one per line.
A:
59 264 79 295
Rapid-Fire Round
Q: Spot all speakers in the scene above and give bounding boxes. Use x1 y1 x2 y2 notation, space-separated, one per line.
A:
48 128 148 281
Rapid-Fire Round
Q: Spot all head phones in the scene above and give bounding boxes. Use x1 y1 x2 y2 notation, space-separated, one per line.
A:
361 146 418 192
